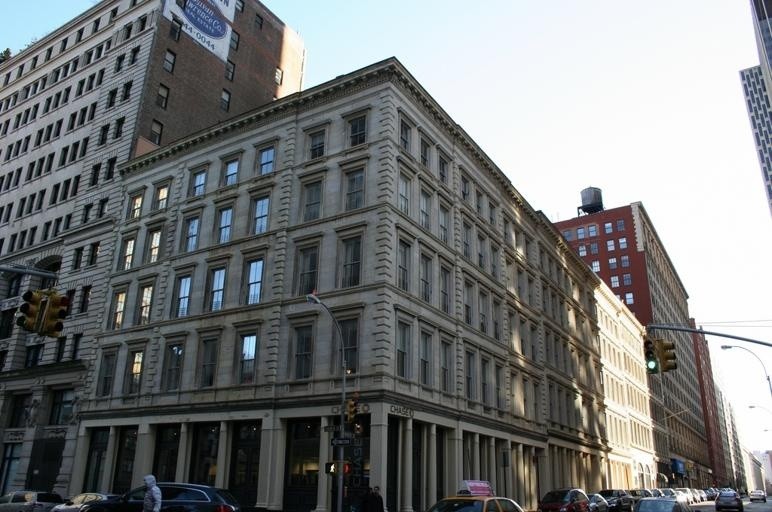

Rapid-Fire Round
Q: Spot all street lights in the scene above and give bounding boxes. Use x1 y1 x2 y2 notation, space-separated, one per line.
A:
747 404 772 415
721 344 772 396
306 294 346 512
763 428 770 432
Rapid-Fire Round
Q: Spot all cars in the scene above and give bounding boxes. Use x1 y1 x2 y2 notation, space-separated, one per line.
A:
1 482 246 511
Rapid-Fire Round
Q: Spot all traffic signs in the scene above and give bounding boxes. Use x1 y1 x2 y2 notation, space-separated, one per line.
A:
344 432 356 438
330 438 351 446
323 425 341 432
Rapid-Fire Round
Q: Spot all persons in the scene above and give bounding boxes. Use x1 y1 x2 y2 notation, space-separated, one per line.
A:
360 486 383 512
143 474 162 511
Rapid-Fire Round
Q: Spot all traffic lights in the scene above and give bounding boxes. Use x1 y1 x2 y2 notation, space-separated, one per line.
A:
643 338 660 374
343 461 351 473
659 338 677 372
38 293 69 338
324 462 337 474
348 399 357 423
15 289 47 334
